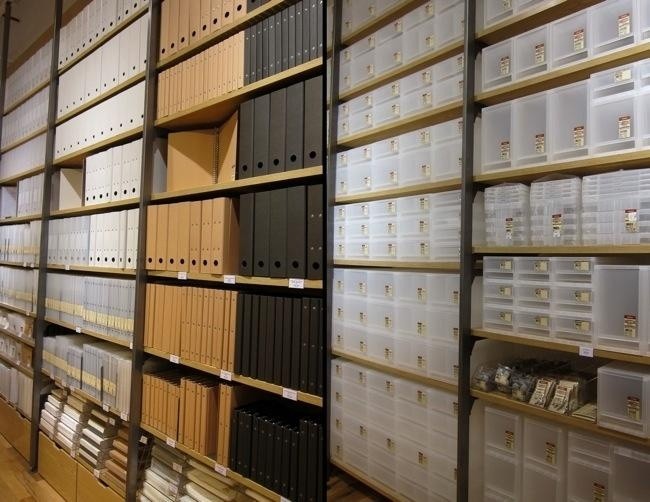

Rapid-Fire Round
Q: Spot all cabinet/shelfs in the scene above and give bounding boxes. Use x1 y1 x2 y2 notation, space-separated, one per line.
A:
0 1 650 502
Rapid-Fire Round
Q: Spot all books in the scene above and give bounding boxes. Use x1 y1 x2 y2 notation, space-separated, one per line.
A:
0 1 329 502
